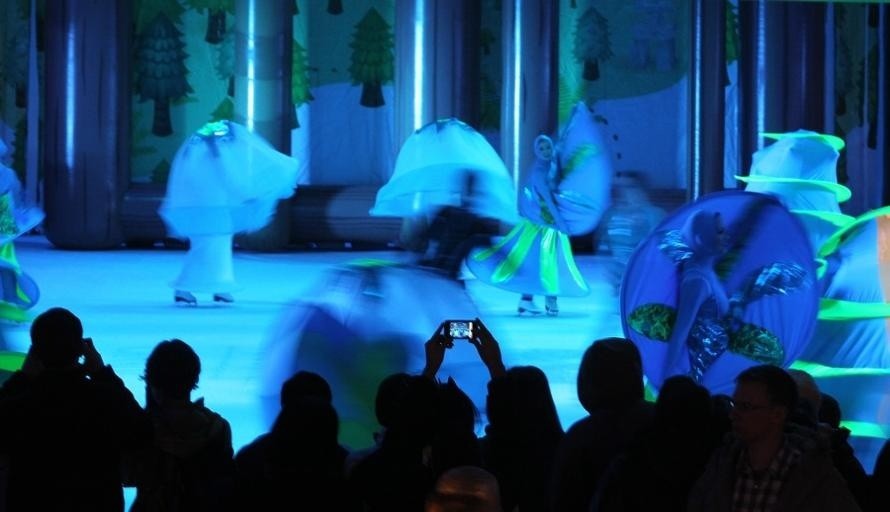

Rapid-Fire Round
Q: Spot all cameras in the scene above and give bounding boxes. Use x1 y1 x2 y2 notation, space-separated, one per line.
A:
444 319 477 341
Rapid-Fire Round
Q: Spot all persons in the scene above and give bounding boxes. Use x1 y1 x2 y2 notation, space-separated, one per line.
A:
466 101 612 315
158 120 299 305
233 319 890 512
399 169 518 383
1 307 234 511
661 189 819 396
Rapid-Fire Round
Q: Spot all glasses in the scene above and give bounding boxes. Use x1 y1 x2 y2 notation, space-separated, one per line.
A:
730 401 770 410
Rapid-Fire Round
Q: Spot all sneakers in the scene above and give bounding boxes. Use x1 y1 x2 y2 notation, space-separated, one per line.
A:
518 297 559 313
214 293 233 302
176 290 196 302
